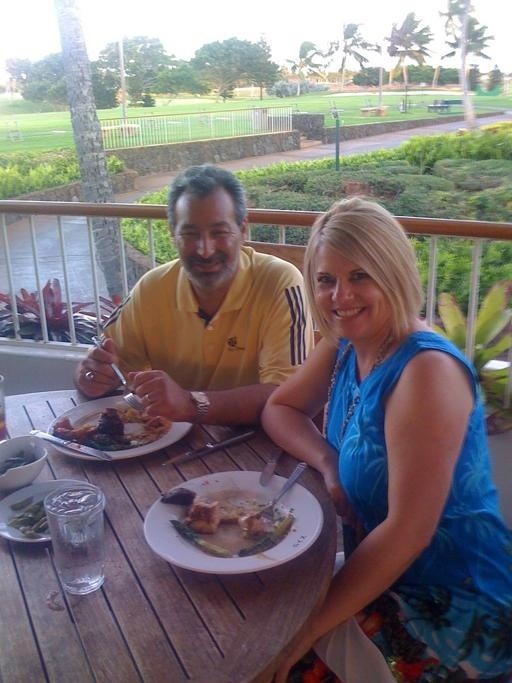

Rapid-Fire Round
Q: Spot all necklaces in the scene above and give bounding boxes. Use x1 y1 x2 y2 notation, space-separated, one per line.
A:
322 330 398 442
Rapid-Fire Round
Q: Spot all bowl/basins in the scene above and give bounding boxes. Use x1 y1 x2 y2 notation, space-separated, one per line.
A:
0 436 50 490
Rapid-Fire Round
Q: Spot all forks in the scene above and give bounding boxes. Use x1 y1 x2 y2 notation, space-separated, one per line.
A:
91 338 141 410
258 462 308 519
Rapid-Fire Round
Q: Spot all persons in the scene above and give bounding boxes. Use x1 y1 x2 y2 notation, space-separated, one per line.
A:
262 194 512 683
72 163 316 427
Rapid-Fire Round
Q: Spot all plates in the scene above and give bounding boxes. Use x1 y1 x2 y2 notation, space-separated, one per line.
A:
143 470 325 575
45 395 192 462
0 480 106 543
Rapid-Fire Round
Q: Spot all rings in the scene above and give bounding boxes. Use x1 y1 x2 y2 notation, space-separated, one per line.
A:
145 393 150 403
85 371 95 383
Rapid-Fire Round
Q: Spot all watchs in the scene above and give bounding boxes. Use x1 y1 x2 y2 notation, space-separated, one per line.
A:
190 389 210 423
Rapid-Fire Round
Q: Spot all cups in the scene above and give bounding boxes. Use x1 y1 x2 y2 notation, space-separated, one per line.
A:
0 376 6 440
46 484 106 596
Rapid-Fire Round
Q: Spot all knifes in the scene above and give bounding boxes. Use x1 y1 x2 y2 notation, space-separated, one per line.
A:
161 430 256 469
260 446 282 485
29 430 113 463
